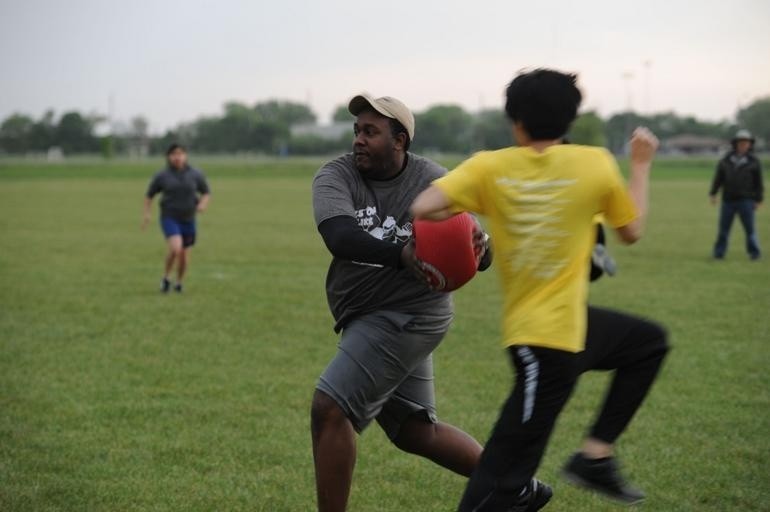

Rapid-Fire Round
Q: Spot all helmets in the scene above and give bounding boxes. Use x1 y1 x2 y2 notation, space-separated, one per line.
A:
731 129 755 145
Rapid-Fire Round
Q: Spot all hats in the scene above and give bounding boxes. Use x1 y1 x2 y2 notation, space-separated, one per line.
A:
348 94 415 142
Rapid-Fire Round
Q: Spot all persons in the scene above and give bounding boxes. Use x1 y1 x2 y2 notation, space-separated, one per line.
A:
143 142 210 292
710 129 763 261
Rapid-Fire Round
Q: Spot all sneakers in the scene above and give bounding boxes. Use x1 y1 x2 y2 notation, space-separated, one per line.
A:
563 451 647 505
508 479 552 512
